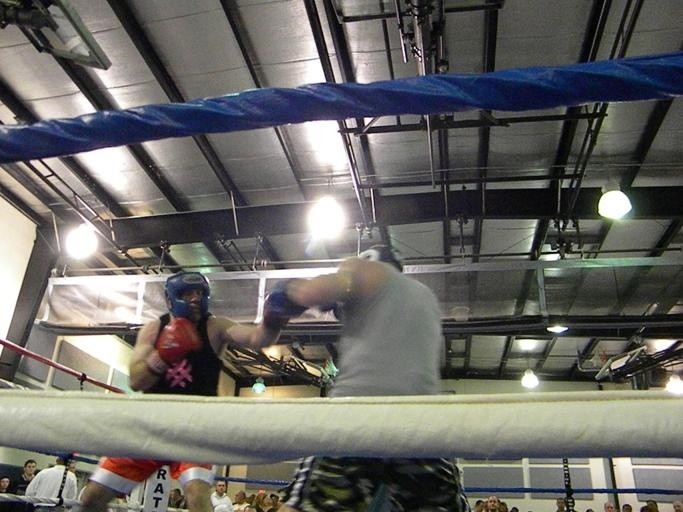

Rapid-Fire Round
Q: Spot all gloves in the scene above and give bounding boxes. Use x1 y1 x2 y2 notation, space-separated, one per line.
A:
145 316 206 377
267 278 309 318
262 290 290 330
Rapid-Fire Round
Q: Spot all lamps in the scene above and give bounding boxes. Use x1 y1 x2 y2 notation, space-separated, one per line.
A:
304 176 347 240
520 349 539 388
665 364 683 394
251 365 266 394
596 174 633 220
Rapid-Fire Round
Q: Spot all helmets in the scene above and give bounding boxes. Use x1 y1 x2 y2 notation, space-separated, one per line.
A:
357 243 403 274
164 270 209 323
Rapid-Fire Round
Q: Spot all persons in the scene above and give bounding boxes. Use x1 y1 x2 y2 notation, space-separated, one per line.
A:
470 493 681 511
74 271 270 511
0 453 286 512
268 243 465 512
